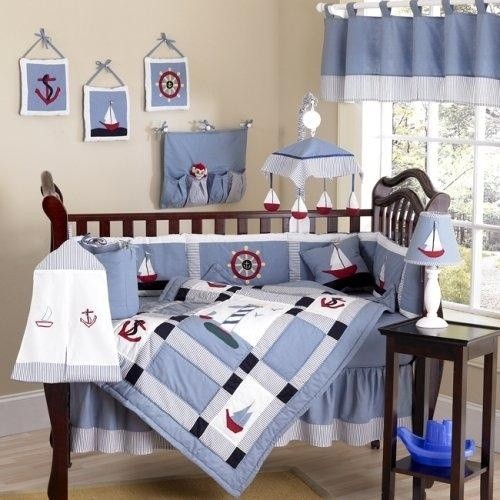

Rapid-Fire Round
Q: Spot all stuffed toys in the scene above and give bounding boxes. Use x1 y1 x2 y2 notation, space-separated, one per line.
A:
190 162 208 180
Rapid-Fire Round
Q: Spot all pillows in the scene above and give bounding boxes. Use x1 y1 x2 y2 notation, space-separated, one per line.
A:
298 235 374 293
78 232 139 319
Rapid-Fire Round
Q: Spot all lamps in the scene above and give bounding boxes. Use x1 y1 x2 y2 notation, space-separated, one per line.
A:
404 211 463 329
296 91 321 199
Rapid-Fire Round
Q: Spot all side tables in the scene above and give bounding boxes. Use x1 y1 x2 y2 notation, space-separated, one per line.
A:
378 316 500 500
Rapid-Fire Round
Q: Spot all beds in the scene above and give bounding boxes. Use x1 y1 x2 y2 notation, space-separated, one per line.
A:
41 168 451 500
